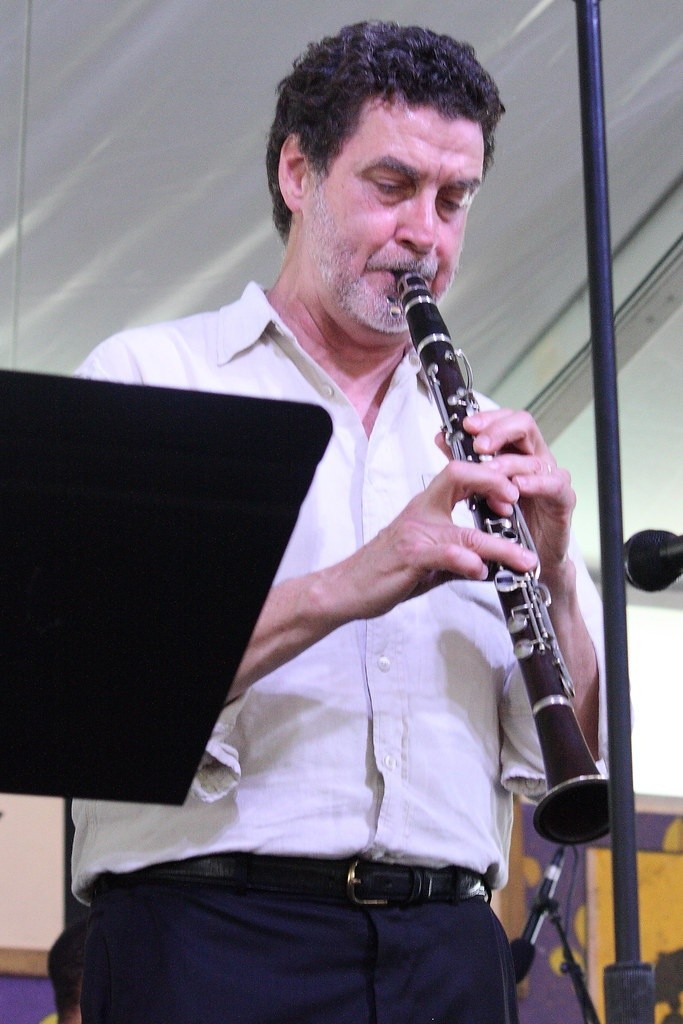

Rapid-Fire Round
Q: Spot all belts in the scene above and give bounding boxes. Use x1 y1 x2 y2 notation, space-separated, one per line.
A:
87 853 485 911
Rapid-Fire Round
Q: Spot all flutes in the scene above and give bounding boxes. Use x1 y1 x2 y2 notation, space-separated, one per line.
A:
384 267 616 846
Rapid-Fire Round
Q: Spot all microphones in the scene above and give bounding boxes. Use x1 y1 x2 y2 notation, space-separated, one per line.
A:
623 529 683 593
510 847 566 982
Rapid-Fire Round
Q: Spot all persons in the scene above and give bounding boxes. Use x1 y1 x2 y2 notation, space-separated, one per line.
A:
49 917 83 1024
67 16 605 1024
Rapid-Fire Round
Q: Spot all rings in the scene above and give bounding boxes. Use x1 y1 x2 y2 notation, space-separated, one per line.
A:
540 459 553 474
